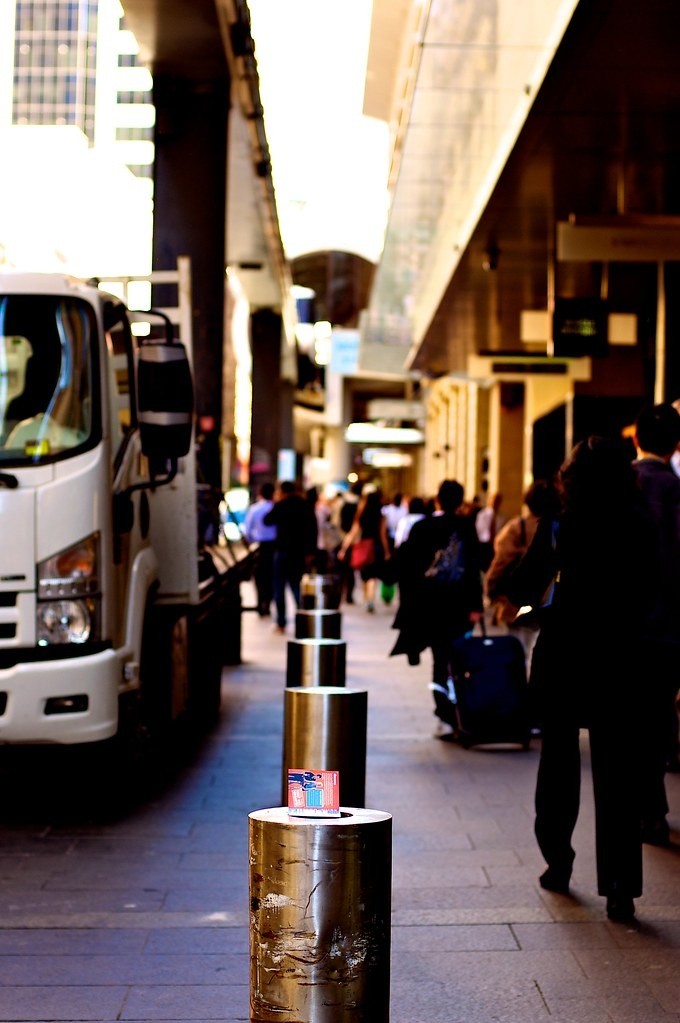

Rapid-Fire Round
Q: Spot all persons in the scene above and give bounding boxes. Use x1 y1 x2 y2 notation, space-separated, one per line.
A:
629 409 680 847
488 426 660 917
240 476 551 754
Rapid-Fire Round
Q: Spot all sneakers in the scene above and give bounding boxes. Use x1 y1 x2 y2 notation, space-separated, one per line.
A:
432 716 453 738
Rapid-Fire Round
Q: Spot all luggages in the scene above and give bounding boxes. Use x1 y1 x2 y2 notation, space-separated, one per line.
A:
448 616 529 750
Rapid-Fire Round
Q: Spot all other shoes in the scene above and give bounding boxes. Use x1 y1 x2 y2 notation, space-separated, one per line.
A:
643 816 671 847
540 865 573 894
366 599 375 613
605 897 635 920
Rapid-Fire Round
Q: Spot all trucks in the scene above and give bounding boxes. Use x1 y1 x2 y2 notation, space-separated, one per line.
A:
0 255 248 823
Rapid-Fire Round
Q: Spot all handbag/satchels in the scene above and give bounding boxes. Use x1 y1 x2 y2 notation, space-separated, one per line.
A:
350 539 375 568
